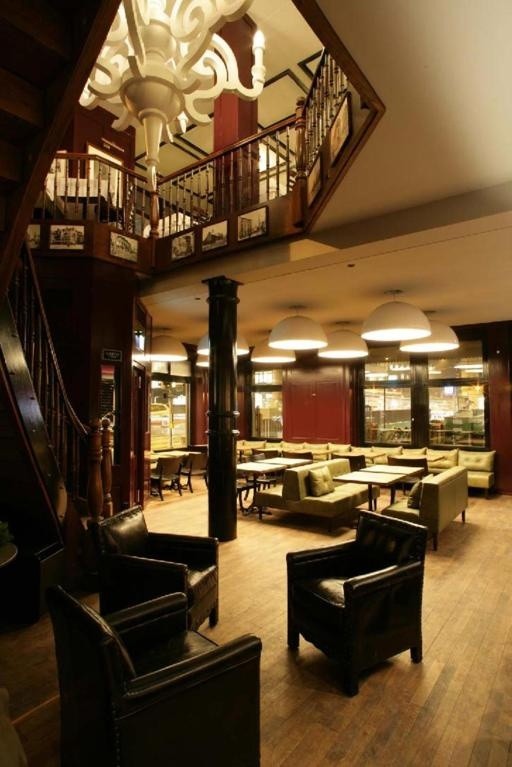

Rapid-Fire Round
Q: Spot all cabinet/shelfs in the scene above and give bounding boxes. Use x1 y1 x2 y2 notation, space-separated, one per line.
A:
279 362 360 446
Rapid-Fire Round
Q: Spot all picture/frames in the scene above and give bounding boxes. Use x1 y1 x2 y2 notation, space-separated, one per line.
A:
84 141 126 211
302 149 326 209
326 86 354 169
235 205 270 244
108 229 143 265
47 223 88 254
169 229 197 262
200 218 230 255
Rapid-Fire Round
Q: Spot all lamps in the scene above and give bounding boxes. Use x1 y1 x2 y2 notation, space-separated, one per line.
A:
76 0 270 196
195 330 249 357
250 329 297 364
317 320 369 361
267 304 330 351
195 356 211 370
399 309 461 354
151 327 188 364
359 289 433 343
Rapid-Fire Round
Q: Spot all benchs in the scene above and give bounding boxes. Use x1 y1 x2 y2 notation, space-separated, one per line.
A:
143 439 499 552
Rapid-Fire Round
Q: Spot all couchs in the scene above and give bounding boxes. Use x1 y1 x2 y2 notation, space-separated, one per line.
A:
95 504 222 632
42 580 266 767
283 509 431 698
